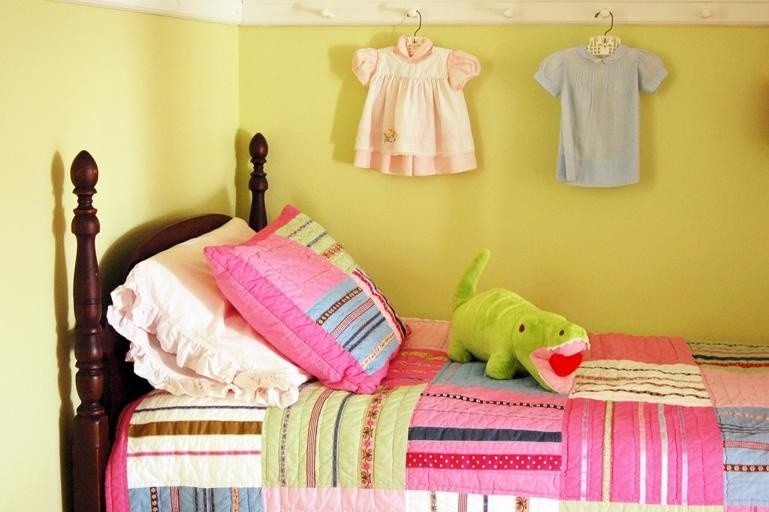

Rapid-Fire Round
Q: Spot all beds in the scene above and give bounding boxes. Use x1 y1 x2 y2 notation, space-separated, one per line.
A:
70 133 769 512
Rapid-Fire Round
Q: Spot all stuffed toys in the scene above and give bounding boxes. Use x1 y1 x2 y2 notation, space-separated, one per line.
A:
445 248 590 395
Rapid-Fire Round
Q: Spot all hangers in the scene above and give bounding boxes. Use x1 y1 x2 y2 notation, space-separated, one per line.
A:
587 11 621 56
405 10 423 55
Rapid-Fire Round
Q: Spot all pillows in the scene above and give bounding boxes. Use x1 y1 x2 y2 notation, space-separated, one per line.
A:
106 305 299 411
204 204 412 394
110 217 314 392
